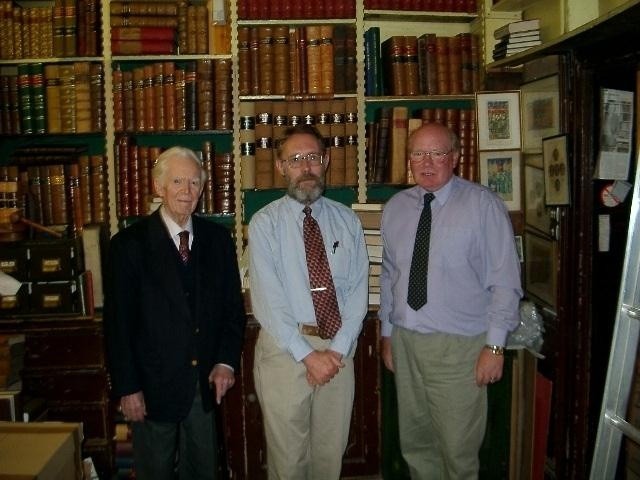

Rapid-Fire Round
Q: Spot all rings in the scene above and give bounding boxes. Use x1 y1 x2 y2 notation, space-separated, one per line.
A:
490 377 496 381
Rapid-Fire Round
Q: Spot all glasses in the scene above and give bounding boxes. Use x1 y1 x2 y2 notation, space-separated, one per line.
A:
409 150 455 162
280 154 324 169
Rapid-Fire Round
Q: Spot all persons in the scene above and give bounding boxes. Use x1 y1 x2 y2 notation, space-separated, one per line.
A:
248 127 369 474
378 122 523 480
103 147 243 476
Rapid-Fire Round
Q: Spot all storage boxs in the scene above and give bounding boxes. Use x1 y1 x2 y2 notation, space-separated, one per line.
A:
23 275 88 317
21 238 83 278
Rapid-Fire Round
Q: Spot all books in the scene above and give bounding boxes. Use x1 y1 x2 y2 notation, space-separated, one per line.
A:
240 1 356 19
349 199 386 307
456 107 477 184
366 27 479 95
1 63 103 135
316 98 359 186
111 0 231 55
197 141 232 212
491 21 541 60
368 2 478 14
290 100 316 130
237 26 356 93
0 142 107 229
111 403 135 475
115 136 161 216
81 225 106 312
2 0 101 58
366 107 419 184
240 100 287 187
114 62 231 132
422 109 455 128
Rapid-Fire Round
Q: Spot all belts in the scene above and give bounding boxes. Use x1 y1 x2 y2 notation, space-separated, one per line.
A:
297 324 325 338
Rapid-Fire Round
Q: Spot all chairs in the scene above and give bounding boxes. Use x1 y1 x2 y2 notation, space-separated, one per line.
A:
0 382 22 422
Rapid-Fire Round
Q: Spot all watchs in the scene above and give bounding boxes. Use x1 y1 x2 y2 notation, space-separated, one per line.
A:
484 345 504 355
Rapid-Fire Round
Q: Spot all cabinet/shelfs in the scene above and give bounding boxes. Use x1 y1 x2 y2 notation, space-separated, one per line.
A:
0 319 112 480
486 1 640 480
0 0 486 294
223 294 381 480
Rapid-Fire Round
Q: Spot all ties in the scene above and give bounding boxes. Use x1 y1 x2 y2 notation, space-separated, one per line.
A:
407 193 436 311
302 207 342 341
177 231 192 262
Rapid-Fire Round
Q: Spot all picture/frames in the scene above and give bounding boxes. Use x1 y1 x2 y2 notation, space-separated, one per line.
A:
514 233 525 264
475 89 523 152
520 70 562 155
542 134 573 207
479 150 525 214
524 163 553 237
524 230 557 307
